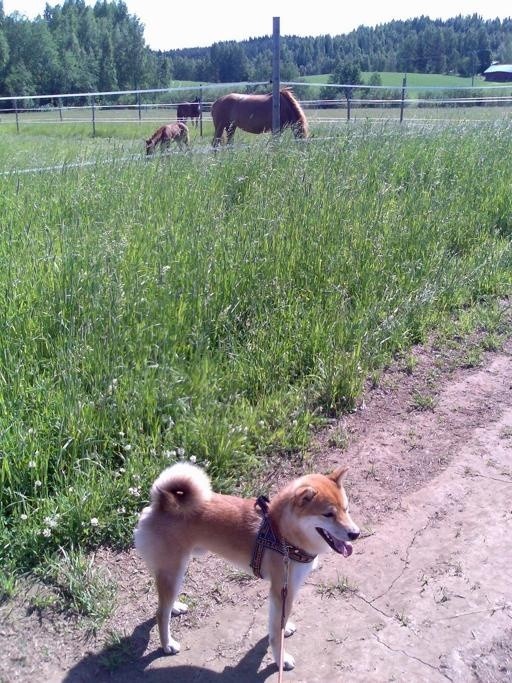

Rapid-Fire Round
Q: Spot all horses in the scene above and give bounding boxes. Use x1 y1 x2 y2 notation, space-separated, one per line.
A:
176 96 202 131
210 83 310 159
144 122 190 160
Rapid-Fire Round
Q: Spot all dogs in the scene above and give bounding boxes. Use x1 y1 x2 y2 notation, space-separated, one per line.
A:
134 460 362 670
